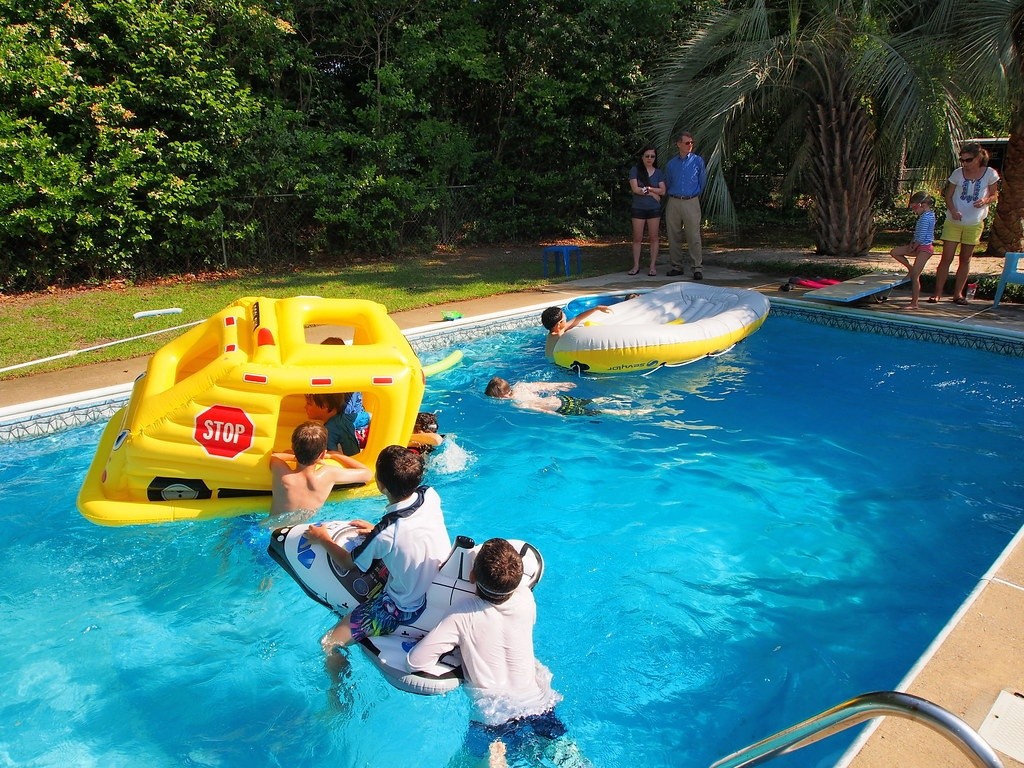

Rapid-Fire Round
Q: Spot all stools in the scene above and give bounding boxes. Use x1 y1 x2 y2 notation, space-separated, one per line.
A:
541 245 582 280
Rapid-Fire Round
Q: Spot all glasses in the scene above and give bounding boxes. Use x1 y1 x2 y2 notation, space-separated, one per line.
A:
912 204 918 210
959 153 979 163
680 141 694 145
642 155 655 158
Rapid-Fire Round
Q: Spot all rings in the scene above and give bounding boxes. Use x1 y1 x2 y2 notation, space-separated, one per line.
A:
980 204 983 206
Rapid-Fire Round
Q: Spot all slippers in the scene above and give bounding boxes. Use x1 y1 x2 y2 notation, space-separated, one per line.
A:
929 296 940 302
648 269 657 276
628 267 640 275
953 298 967 305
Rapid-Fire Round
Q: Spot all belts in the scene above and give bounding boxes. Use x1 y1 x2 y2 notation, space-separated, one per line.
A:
669 193 698 200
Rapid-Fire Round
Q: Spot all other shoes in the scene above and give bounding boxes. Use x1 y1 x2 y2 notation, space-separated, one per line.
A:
693 271 703 280
665 269 684 276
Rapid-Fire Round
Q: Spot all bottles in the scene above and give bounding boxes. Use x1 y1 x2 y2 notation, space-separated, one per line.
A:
965 284 978 300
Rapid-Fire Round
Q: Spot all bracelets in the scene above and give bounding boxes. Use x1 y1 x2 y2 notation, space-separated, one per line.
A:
643 187 647 195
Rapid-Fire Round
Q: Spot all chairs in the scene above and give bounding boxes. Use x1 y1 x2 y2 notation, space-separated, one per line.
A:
993 250 1023 307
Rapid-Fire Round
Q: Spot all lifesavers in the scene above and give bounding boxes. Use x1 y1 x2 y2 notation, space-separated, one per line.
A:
423 348 463 377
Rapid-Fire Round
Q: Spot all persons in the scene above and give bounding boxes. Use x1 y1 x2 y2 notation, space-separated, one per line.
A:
485 379 519 399
625 293 639 301
304 393 361 457
890 191 937 310
320 337 371 448
663 130 707 280
406 538 542 701
407 413 444 456
929 143 1000 305
303 444 452 646
542 306 614 358
268 419 373 518
628 146 666 276
